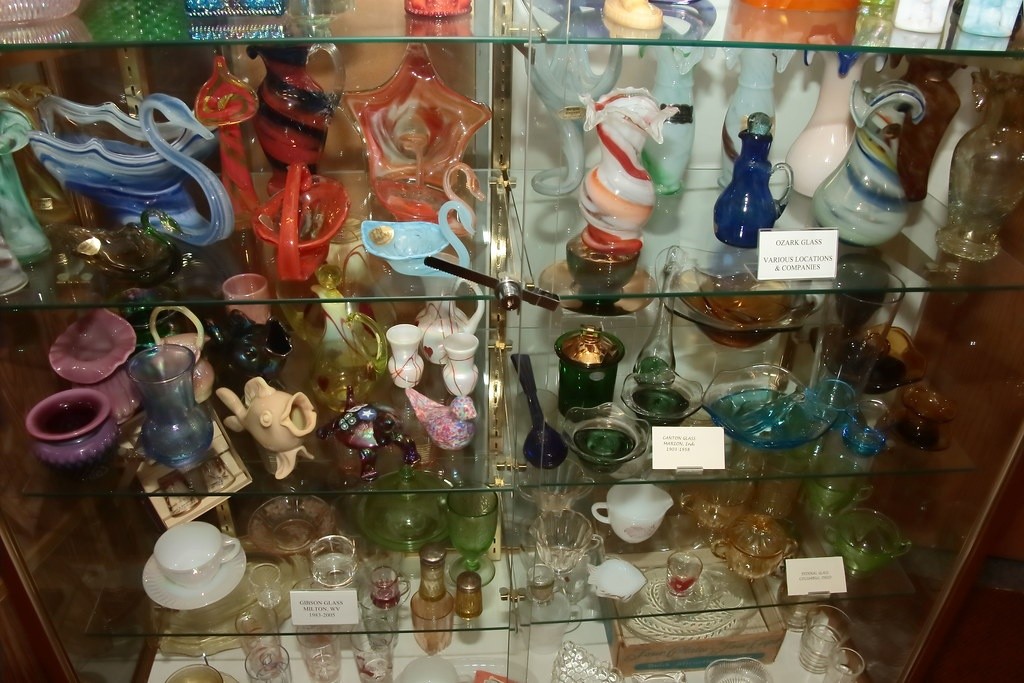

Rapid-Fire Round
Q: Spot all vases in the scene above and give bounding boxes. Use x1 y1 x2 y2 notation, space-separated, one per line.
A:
195 41 347 230
25 287 213 474
577 21 1024 261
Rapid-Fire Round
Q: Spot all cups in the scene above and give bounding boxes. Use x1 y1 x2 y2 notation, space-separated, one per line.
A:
678 482 799 581
665 552 703 596
527 564 554 606
777 582 865 683
591 478 674 543
553 321 626 421
804 262 911 426
234 533 472 683
805 455 912 579
153 521 240 588
165 664 223 683
530 508 603 574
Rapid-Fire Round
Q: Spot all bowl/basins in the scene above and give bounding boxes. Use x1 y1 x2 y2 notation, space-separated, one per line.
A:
565 233 642 291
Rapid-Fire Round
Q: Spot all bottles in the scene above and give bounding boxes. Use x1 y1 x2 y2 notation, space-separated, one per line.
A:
576 42 1023 263
386 324 424 389
442 334 479 397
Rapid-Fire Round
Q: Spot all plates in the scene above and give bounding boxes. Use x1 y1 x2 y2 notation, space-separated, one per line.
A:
537 260 659 316
142 533 246 611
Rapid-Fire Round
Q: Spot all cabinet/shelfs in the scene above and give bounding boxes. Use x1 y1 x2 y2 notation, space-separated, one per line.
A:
0 0 1024 683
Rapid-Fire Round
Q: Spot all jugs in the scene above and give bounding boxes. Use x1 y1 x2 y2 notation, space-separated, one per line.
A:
413 253 485 365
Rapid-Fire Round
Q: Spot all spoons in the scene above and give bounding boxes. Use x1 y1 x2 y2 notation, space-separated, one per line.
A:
510 353 568 468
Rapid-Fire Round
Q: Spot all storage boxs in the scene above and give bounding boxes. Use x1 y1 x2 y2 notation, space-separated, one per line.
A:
592 510 787 676
